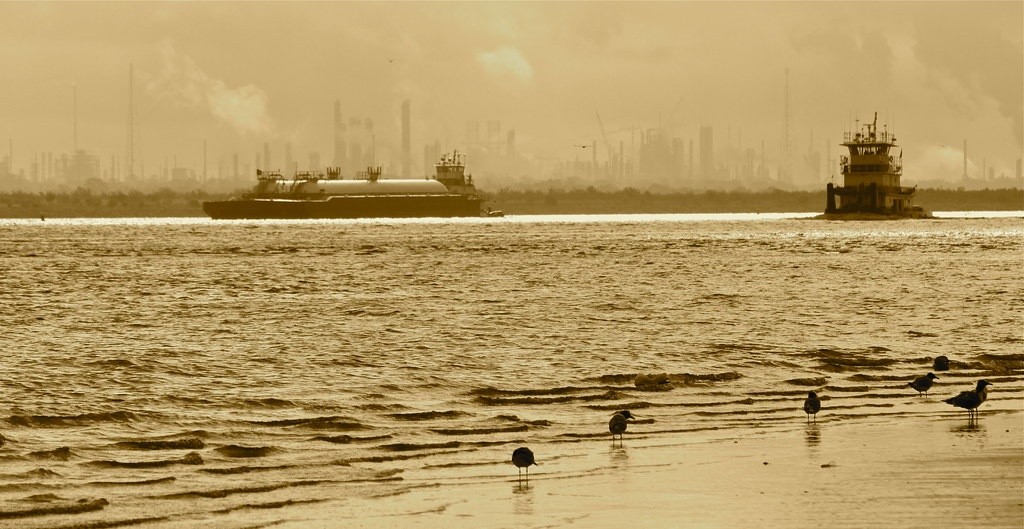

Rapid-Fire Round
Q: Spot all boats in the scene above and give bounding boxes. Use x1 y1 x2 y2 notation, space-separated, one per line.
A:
817 109 929 219
195 149 503 218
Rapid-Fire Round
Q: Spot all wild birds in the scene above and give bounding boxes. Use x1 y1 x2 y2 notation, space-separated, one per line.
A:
804 391 821 418
512 447 538 473
941 379 993 418
908 372 940 395
609 411 635 440
